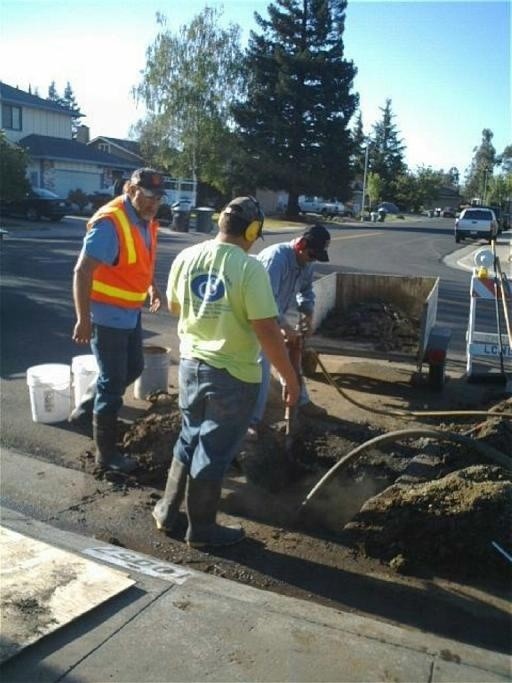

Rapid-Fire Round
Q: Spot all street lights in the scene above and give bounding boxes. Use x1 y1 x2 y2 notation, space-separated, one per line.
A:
358 131 372 220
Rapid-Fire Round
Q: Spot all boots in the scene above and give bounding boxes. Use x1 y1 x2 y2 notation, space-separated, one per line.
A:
184 472 246 548
150 458 189 533
66 384 95 437
92 412 137 473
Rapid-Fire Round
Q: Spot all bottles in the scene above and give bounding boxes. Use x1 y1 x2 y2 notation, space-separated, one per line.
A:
480 266 488 278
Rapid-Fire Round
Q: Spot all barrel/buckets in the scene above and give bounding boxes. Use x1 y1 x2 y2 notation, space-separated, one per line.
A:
26 363 71 424
72 355 101 409
134 346 171 401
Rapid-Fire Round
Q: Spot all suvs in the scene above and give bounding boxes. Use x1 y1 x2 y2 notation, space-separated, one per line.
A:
293 195 345 217
453 208 498 243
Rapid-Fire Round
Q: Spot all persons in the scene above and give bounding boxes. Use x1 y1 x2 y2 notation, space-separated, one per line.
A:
244 221 331 435
151 194 301 548
67 168 164 475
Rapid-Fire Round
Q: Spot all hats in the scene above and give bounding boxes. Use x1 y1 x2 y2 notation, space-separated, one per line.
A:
223 195 265 242
302 223 331 263
130 167 169 198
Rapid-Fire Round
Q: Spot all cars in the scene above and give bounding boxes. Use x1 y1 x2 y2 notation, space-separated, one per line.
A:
422 206 453 218
376 202 400 215
0 188 70 221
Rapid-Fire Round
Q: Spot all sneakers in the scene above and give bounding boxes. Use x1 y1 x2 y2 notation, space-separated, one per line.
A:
300 401 328 417
